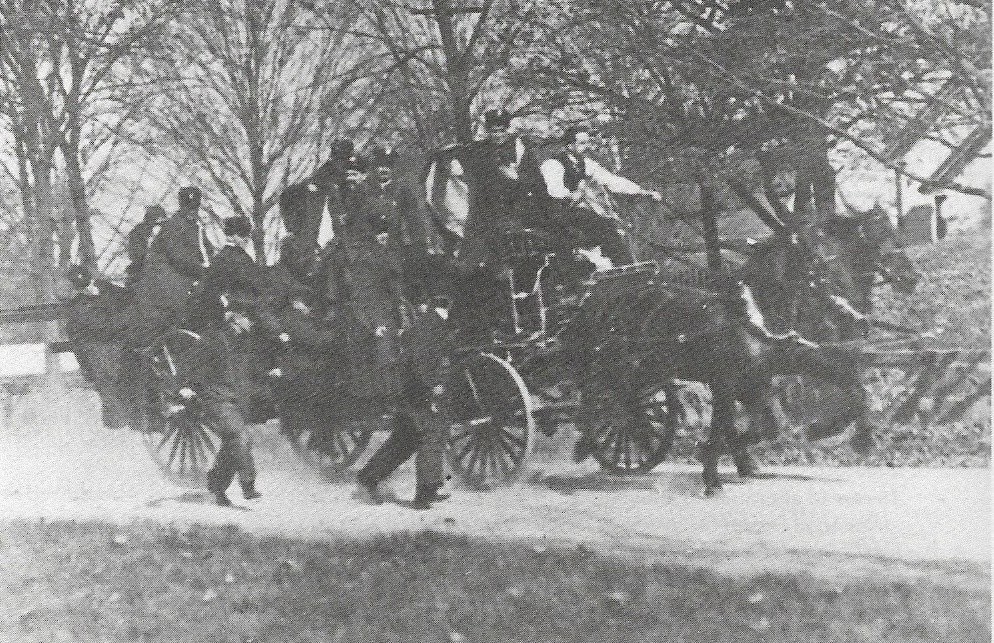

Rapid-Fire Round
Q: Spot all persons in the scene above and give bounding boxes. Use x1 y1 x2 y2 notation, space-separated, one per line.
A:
71 105 669 512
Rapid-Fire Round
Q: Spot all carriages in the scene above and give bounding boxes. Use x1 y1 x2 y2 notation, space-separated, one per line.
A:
136 197 930 503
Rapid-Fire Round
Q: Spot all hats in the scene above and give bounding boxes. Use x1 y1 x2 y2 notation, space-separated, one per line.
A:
484 109 513 125
222 216 252 236
68 266 91 288
374 149 399 166
345 154 367 174
180 187 202 208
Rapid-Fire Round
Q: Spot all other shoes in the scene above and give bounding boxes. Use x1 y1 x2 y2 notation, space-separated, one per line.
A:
241 484 260 499
208 485 233 506
358 469 384 506
412 489 451 509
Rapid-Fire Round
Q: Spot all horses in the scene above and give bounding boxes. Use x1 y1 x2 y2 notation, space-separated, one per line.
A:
569 208 922 499
